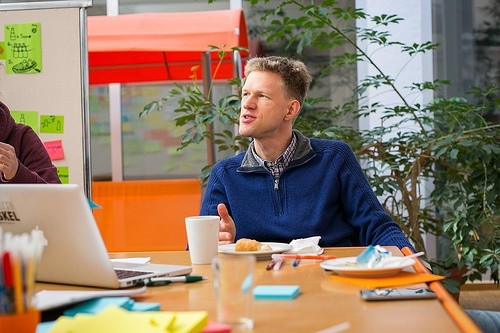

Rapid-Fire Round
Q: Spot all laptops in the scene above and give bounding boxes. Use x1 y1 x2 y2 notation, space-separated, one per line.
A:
0 184 193 288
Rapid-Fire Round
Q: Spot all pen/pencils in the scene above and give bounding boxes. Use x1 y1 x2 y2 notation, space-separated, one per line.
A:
274 258 283 270
137 276 208 284
0 224 50 315
293 258 301 267
266 259 274 270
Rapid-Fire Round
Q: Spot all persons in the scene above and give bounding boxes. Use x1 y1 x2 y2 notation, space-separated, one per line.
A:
187 55 436 274
0 101 62 184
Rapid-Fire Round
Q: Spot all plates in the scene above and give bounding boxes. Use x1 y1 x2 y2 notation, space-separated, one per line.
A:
216 242 291 260
321 255 416 278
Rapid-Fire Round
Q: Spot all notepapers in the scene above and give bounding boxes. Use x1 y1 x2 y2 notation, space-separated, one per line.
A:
253 283 300 300
32 295 234 333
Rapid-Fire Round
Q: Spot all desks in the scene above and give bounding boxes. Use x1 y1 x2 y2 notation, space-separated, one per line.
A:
0 243 468 333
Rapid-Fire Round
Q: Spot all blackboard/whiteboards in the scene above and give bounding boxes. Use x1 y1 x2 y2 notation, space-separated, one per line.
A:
0 3 92 203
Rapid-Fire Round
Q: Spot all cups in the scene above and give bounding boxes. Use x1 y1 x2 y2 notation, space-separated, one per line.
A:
210 255 254 331
185 216 220 264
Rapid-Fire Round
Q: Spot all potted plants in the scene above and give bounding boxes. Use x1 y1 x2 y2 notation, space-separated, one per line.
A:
135 0 499 302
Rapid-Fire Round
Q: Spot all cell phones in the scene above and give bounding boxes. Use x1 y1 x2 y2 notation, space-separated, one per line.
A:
359 287 436 300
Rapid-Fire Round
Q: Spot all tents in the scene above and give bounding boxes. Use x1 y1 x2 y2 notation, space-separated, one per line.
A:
88 8 250 180
